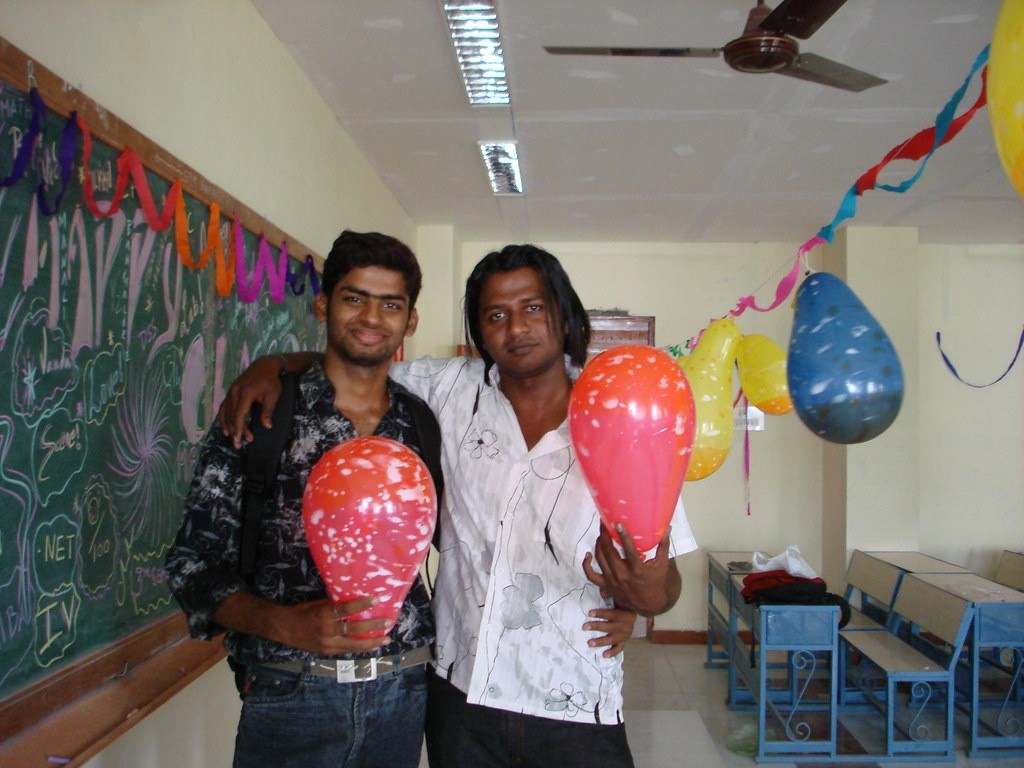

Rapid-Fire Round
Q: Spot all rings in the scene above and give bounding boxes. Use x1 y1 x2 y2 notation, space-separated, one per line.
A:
333 605 339 618
342 621 348 637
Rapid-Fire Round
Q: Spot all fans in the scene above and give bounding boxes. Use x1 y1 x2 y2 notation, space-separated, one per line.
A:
542 0 892 97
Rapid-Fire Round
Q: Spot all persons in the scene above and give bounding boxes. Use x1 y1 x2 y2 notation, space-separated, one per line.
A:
163 229 698 768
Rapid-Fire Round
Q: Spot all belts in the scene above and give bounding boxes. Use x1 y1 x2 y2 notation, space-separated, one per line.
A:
257 642 433 684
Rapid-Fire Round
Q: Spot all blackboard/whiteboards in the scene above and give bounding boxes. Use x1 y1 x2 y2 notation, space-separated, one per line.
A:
1 38 327 768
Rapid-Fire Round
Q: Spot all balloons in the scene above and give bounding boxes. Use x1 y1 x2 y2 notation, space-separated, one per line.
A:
301 433 438 643
566 342 697 561
785 268 906 445
672 315 794 483
983 0 1023 197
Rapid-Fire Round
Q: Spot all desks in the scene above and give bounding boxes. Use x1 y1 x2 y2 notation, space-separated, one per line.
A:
845 549 1024 759
706 548 838 763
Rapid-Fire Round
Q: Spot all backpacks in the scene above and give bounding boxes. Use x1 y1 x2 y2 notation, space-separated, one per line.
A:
741 569 831 604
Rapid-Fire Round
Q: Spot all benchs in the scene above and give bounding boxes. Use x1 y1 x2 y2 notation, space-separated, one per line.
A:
833 572 976 763
831 547 905 686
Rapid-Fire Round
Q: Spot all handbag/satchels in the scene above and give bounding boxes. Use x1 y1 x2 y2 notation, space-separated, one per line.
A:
754 544 818 579
